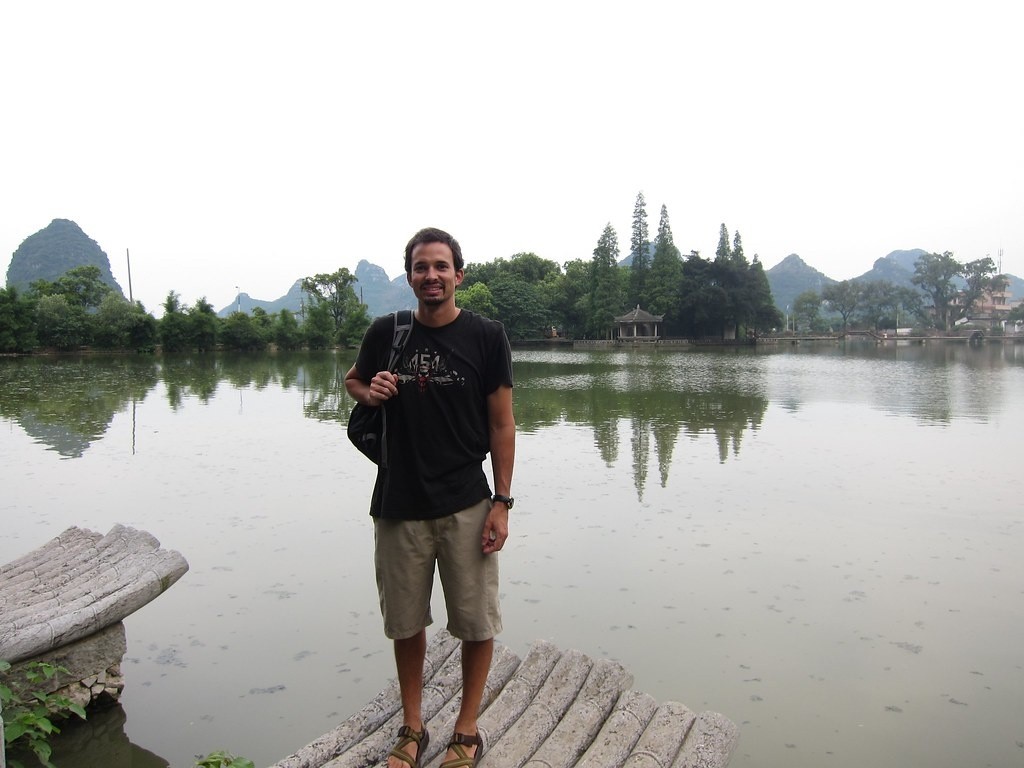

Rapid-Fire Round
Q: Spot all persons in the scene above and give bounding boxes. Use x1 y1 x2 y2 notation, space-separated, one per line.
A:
344 227 519 766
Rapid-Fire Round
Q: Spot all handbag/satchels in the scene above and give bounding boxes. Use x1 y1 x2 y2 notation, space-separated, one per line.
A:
348 395 386 465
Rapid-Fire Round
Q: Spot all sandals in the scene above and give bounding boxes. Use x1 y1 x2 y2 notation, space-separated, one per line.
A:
441 730 485 768
389 724 432 768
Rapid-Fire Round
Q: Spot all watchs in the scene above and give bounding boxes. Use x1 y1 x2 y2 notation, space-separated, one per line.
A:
493 494 515 509
